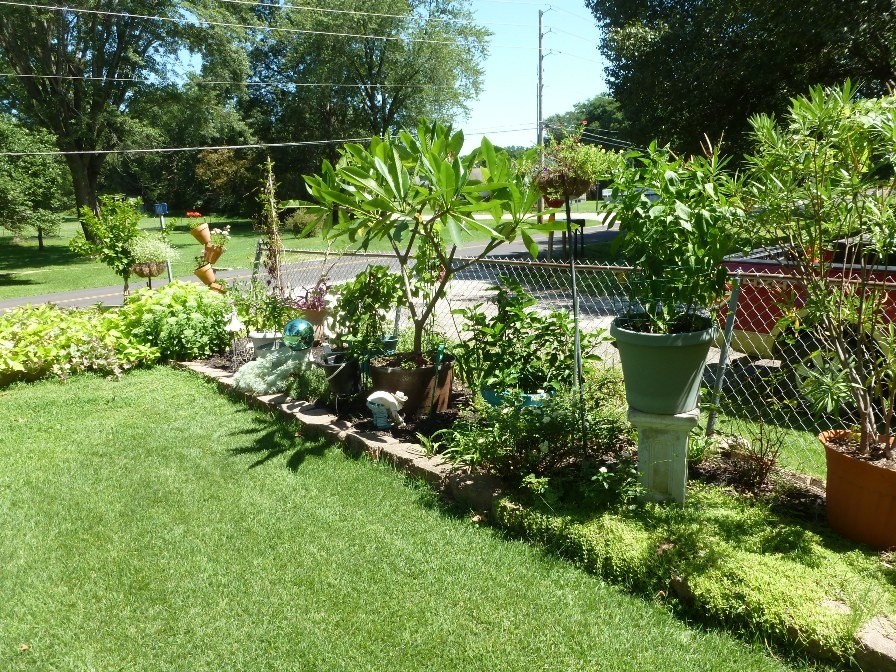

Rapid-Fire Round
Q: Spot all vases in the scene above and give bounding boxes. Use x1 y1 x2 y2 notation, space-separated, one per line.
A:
543 195 565 208
133 263 166 278
194 263 215 285
566 177 592 200
191 223 211 244
204 244 224 264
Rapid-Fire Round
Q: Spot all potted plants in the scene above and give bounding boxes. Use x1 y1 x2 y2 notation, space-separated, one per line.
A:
604 78 896 550
240 116 579 419
210 279 229 295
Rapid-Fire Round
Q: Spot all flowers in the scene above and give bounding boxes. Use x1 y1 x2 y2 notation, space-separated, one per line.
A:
514 120 624 201
129 230 184 265
187 211 232 268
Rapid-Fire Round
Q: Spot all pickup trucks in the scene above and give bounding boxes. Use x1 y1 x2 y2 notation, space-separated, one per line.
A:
713 238 895 419
